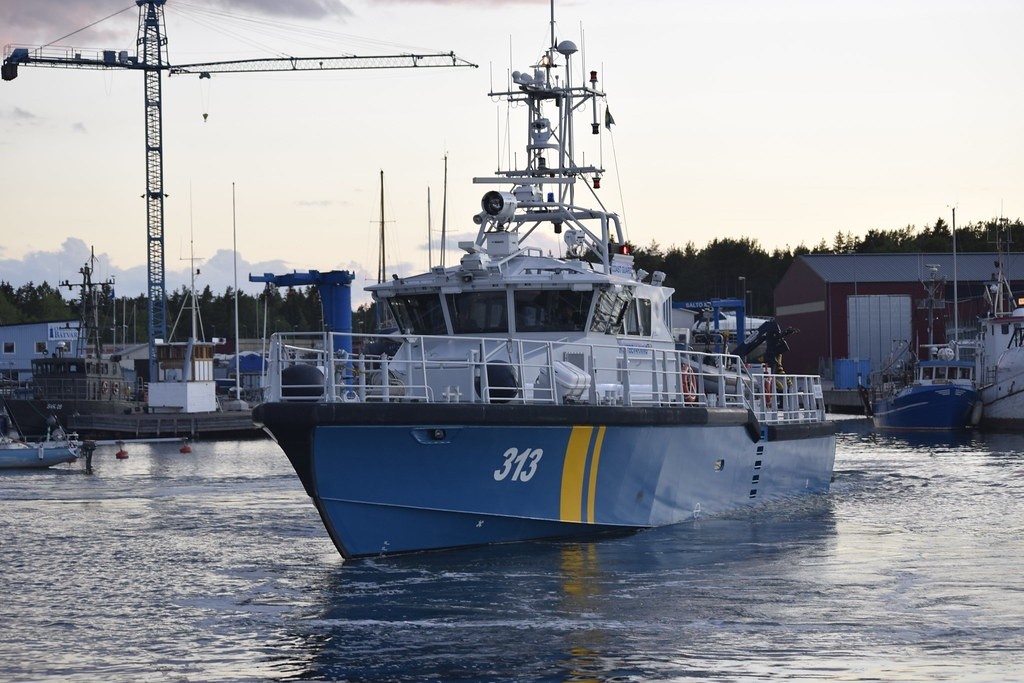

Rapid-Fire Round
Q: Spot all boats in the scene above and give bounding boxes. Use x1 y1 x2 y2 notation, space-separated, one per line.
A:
968 226 1024 431
246 1 838 565
856 262 984 434
0 377 81 470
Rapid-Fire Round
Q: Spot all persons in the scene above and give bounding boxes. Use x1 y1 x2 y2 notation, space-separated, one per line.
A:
774 367 793 409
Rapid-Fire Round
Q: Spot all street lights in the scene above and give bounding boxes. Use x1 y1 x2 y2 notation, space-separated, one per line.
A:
738 276 746 334
746 290 752 334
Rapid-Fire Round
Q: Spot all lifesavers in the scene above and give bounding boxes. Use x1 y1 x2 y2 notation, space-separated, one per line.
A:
680 362 697 403
763 374 774 407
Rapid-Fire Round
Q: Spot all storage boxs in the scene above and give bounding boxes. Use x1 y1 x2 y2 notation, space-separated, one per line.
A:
834 359 872 390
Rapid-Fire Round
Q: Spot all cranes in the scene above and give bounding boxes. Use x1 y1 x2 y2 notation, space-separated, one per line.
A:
0 1 479 377
